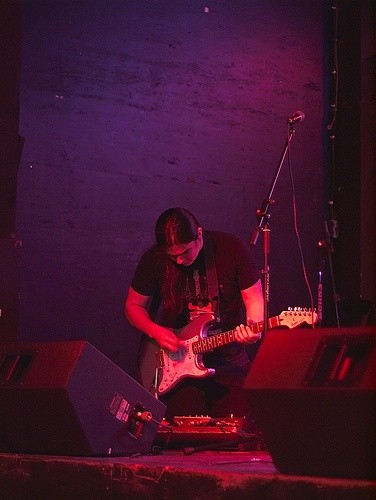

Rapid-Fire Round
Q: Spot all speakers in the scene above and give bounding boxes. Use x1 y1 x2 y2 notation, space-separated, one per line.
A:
0 340 167 457
241 328 375 483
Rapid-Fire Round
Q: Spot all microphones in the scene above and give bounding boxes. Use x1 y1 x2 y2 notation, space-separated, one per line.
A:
286 111 305 125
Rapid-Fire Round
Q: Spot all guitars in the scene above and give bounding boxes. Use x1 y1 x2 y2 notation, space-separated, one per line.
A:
139 306 318 399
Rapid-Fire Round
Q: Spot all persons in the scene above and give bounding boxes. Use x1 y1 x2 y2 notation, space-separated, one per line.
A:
124 207 262 416
